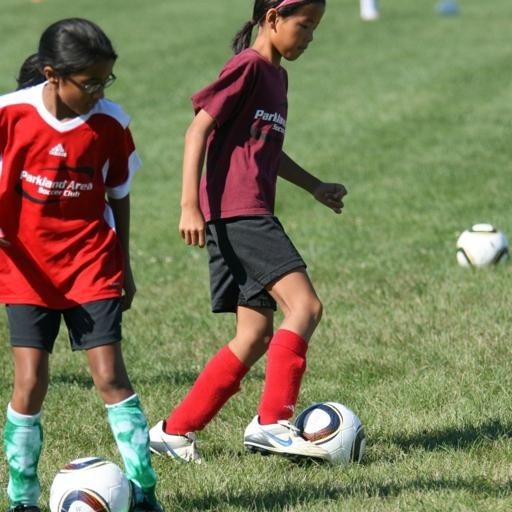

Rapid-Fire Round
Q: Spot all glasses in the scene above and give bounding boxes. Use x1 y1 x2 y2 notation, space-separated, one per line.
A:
66 72 116 94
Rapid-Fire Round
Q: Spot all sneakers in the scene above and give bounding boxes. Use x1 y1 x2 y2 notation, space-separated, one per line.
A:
244 415 332 464
149 420 203 466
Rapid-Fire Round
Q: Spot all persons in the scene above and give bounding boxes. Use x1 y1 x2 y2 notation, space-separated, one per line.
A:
149 0 348 466
0 17 166 512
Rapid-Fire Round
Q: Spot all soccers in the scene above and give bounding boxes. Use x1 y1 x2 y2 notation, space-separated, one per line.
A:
296 401 365 464
457 223 508 266
50 457 131 512
